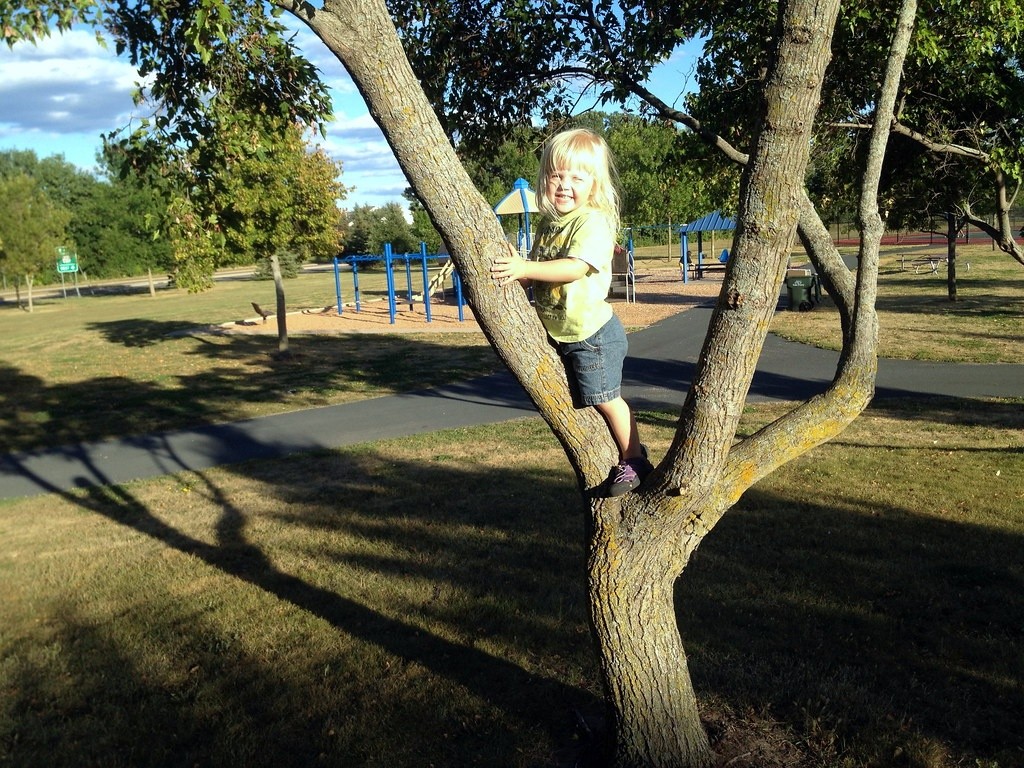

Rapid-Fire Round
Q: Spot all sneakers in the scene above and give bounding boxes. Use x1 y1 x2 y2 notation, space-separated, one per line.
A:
610 454 654 496
640 444 647 459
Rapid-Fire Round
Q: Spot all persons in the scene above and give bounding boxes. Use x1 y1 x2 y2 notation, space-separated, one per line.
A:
490 128 653 498
679 251 695 280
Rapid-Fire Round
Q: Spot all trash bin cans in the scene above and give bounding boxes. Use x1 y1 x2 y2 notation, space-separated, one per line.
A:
787 274 822 312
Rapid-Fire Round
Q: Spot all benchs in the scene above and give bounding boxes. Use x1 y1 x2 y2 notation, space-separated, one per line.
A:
897 259 938 261
695 264 727 280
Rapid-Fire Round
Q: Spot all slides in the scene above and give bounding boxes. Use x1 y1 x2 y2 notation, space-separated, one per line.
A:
412 260 455 301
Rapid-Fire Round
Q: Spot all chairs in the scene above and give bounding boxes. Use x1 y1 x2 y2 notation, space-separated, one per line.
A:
251 302 275 324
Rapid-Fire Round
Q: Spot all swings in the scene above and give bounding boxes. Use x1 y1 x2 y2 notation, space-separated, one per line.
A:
641 231 653 264
660 230 672 263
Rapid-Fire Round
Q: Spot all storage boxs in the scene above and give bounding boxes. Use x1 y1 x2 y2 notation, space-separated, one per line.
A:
612 249 628 273
788 269 811 277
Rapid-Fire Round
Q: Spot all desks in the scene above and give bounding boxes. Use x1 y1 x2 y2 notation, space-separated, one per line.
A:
895 253 962 275
